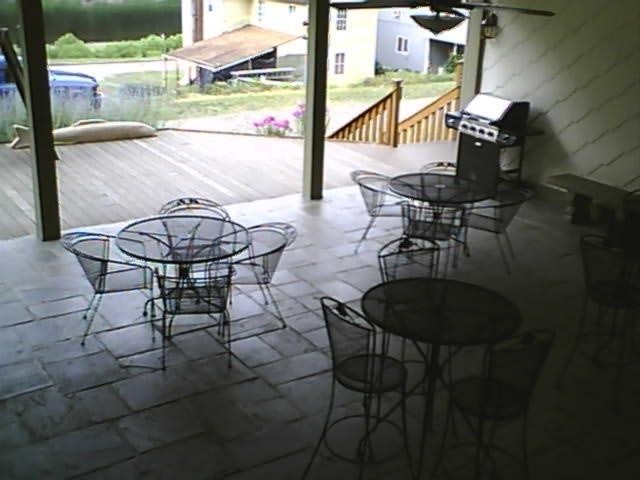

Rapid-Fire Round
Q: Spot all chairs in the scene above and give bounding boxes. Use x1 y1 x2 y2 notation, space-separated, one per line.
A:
300 296 414 480
554 234 639 416
152 265 236 374
432 328 556 479
60 231 159 349
350 168 535 278
376 236 451 395
157 196 236 306
204 221 298 345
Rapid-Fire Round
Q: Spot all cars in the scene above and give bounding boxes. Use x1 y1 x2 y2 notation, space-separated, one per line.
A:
0 56 101 141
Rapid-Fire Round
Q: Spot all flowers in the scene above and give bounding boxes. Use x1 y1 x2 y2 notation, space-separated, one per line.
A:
252 103 306 137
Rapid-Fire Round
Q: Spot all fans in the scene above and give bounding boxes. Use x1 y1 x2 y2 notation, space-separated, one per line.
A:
327 0 556 35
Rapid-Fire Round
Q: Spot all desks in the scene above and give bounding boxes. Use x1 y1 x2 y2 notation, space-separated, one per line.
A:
114 214 254 345
357 276 522 480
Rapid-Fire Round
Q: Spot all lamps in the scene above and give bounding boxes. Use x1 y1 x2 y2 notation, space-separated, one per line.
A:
481 9 502 38
413 15 464 34
